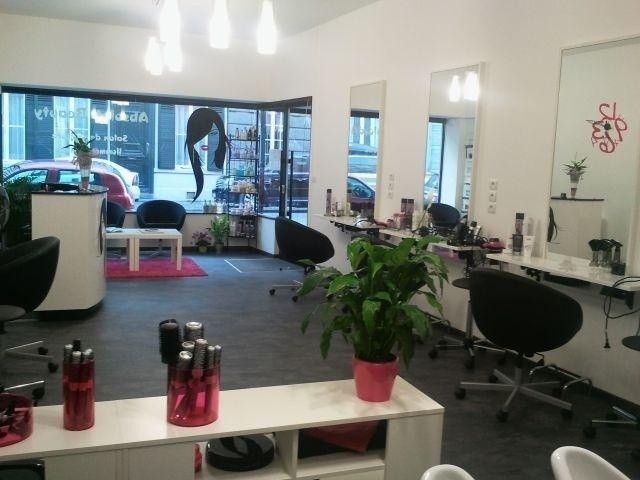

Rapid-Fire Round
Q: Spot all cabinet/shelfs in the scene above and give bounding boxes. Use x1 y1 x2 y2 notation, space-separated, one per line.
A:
1 415 445 480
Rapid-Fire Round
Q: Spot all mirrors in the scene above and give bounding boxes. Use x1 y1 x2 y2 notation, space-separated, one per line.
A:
544 35 640 278
423 63 482 220
344 79 383 223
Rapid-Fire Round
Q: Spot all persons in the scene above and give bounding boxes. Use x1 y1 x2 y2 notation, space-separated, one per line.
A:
184 108 233 205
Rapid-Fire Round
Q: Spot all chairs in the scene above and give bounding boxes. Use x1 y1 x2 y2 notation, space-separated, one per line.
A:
0 236 60 372
107 199 186 259
269 215 334 302
455 266 583 422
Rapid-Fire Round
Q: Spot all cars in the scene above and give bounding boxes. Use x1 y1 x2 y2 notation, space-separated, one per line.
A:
50 156 140 202
3 159 135 213
213 168 441 210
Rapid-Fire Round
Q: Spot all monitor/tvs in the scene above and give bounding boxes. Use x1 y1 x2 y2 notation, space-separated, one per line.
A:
45 169 60 183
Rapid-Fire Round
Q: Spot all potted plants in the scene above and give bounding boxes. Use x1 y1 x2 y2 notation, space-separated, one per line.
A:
63 129 96 190
561 153 589 197
289 233 454 402
190 214 231 253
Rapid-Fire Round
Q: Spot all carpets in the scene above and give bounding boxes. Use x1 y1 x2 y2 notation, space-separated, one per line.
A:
104 258 208 279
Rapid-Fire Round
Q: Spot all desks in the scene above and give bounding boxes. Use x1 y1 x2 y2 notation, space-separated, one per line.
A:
107 229 182 272
